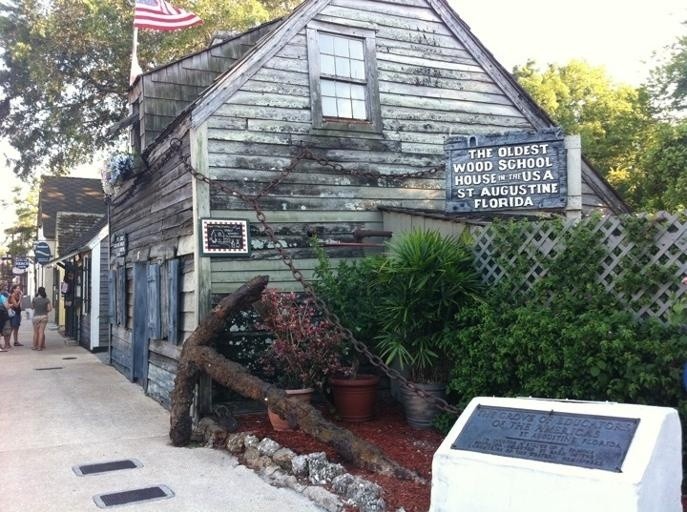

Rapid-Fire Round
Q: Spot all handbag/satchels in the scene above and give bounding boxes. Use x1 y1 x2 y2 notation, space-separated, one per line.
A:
7 309 15 318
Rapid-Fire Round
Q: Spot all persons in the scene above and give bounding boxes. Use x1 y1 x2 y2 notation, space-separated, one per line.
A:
30 286 51 351
34 293 45 347
0 337 8 352
0 279 16 348
8 284 25 346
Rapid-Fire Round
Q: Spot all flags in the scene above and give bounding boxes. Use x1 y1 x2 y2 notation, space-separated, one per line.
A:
131 1 203 32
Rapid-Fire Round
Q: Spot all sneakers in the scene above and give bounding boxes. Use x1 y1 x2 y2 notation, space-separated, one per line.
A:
0 342 45 352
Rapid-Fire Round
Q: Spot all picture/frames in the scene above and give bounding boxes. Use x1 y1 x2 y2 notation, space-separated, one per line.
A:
199 216 252 258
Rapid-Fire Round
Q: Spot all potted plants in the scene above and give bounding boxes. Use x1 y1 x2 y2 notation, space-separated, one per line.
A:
308 223 492 430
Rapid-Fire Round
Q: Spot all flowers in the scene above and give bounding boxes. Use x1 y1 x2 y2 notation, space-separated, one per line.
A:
100 148 134 196
248 282 353 391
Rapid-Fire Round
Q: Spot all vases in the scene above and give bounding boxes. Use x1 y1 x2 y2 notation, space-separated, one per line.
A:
266 389 314 433
